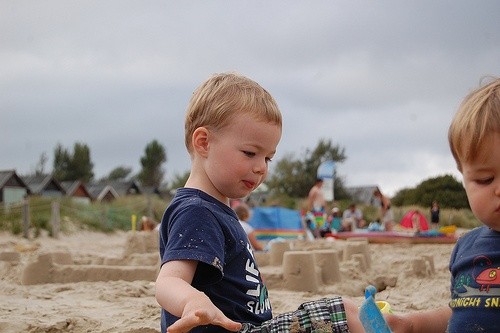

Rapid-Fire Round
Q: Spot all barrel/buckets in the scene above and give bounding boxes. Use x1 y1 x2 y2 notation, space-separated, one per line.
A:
374 300 393 313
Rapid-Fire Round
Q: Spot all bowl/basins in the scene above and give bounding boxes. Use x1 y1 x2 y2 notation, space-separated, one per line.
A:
357 285 394 333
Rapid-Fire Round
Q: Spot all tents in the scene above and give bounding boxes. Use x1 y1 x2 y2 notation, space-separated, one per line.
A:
246 207 313 247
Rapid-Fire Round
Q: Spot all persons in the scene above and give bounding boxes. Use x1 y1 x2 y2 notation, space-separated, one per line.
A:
141 178 441 251
155 73 366 333
381 76 500 333
